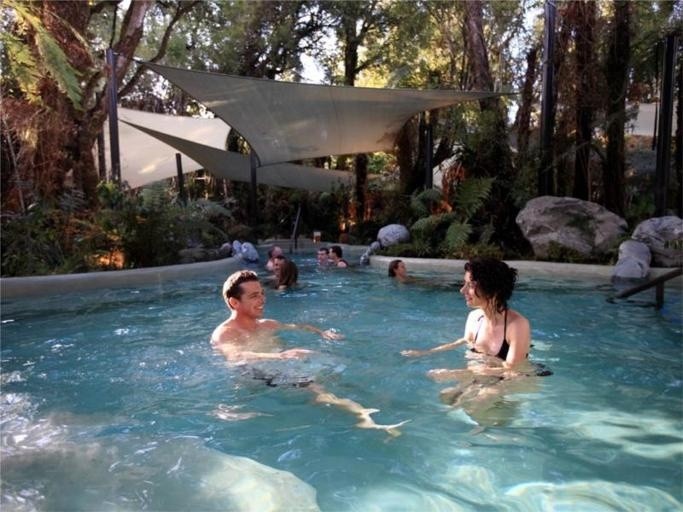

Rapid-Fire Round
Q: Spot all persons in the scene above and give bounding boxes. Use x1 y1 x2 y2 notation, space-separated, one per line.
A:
328 245 347 268
263 245 284 272
261 259 298 293
209 268 413 439
397 254 553 436
387 259 464 292
263 255 286 282
316 248 333 264
